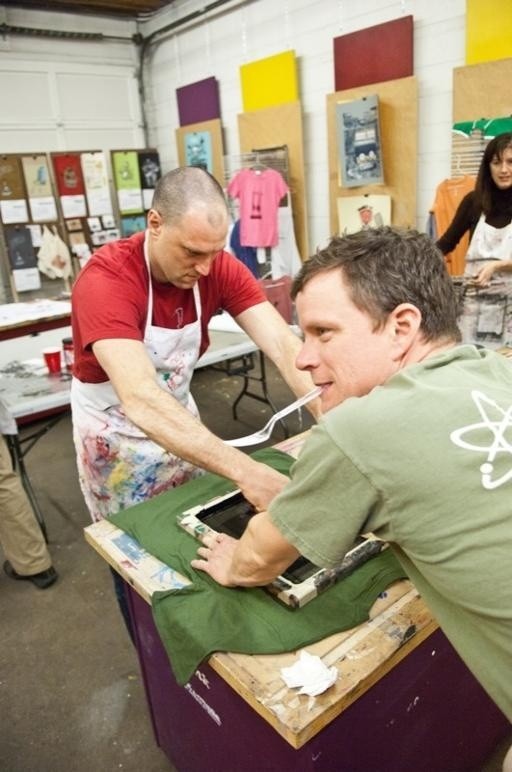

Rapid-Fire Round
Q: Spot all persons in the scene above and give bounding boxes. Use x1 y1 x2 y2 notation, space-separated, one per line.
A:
68 165 328 520
2 391 56 587
426 135 512 287
190 228 510 772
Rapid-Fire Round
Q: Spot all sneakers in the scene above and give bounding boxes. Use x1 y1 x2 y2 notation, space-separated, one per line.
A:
3 561 58 589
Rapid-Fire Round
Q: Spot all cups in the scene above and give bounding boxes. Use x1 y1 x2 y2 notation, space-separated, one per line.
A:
43 350 62 378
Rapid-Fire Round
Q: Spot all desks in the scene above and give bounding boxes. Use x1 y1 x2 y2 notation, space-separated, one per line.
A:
82 431 511 772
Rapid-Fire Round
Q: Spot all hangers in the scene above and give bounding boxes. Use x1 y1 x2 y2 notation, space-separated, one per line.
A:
249 151 268 169
447 154 468 177
287 183 298 196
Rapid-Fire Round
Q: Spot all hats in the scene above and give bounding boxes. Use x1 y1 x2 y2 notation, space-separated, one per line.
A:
358 206 373 212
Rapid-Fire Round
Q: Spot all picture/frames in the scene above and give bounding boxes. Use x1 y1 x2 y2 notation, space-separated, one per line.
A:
176 487 389 610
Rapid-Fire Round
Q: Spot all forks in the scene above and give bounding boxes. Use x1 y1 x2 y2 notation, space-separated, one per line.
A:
220 386 324 447
